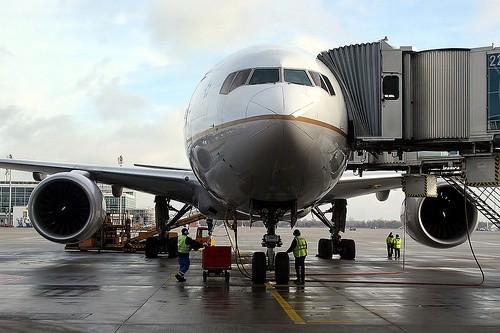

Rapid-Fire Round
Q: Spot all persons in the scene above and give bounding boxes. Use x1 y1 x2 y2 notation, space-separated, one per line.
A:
175 228 203 282
393 235 401 258
386 232 394 257
286 230 307 284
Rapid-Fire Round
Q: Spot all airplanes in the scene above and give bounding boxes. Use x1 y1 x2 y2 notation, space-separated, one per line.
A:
0 37 500 285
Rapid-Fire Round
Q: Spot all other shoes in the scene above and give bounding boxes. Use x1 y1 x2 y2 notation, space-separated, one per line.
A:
294 279 306 284
175 274 187 282
